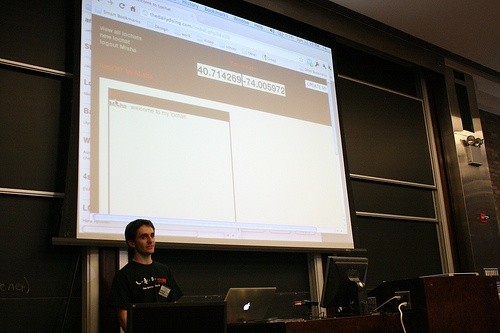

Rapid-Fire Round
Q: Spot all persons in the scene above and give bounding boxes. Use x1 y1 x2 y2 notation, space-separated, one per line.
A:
107 220 184 333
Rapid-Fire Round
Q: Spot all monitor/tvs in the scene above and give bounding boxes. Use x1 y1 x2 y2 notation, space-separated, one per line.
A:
320 255 371 316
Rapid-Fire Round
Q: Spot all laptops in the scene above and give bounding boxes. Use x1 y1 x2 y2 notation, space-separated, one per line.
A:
224 286 277 325
